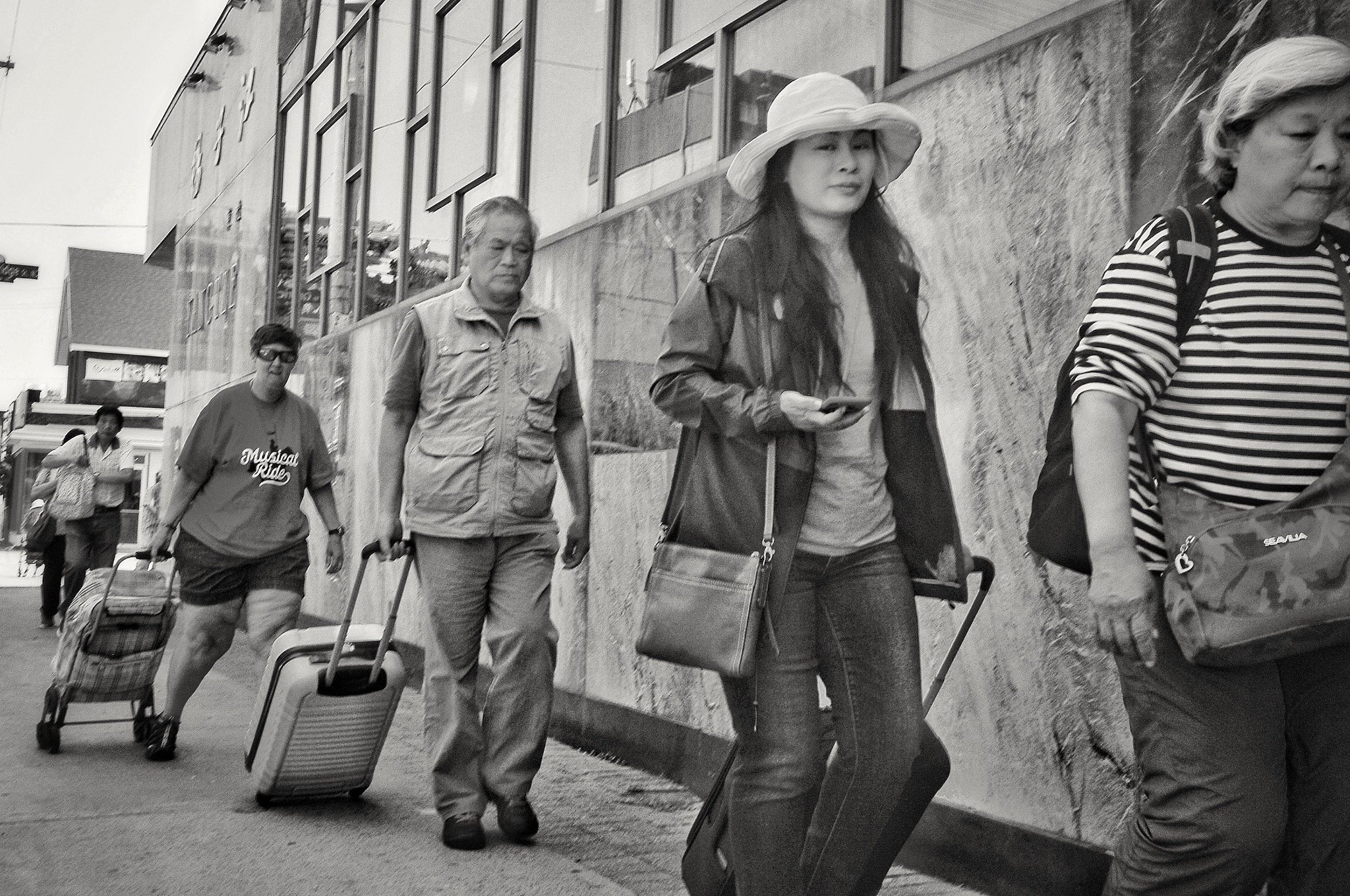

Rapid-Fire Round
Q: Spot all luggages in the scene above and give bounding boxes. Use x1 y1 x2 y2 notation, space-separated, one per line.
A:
244 541 416 809
36 550 182 753
681 555 995 896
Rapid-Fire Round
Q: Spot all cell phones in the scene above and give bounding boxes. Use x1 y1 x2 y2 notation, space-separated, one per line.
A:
819 397 873 415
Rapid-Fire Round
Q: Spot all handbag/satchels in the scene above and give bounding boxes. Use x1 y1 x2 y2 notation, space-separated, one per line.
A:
635 542 774 679
1157 437 1350 666
48 433 98 520
25 513 57 553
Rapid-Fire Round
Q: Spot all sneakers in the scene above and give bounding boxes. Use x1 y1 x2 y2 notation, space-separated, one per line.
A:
442 817 487 850
496 803 539 838
146 710 182 761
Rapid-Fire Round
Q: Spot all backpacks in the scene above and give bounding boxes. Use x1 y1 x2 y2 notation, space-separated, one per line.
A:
1027 205 1350 575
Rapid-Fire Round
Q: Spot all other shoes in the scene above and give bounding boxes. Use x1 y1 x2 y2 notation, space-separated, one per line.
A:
56 626 62 639
39 623 53 628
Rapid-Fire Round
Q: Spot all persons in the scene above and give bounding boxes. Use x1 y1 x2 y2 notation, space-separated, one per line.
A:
18 405 134 638
649 73 974 896
376 198 592 849
146 323 345 760
1069 35 1350 896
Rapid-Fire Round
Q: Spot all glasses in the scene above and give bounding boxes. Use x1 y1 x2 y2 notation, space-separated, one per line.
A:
258 348 297 363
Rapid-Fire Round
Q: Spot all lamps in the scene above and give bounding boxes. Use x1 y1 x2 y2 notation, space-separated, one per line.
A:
227 0 254 9
181 72 205 89
202 31 228 55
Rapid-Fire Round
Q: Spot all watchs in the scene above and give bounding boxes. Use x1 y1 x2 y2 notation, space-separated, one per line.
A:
329 524 346 536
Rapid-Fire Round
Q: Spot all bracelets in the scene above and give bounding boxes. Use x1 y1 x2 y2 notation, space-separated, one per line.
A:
157 520 178 533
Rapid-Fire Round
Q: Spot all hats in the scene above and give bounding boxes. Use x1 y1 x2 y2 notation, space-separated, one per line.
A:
726 71 922 204
29 499 45 510
